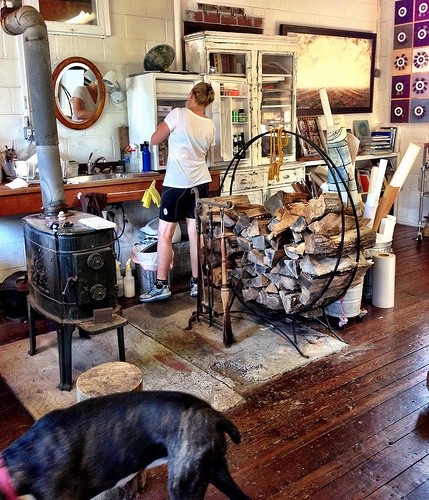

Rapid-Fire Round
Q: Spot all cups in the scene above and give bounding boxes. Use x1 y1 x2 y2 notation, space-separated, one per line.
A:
232 111 238 122
66 161 79 178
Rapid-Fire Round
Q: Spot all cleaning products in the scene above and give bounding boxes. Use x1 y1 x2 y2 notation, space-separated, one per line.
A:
124 141 152 173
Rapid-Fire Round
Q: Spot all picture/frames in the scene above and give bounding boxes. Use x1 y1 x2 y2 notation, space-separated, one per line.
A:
278 23 378 117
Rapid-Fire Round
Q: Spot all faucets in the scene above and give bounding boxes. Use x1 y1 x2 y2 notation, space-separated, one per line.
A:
87 152 107 175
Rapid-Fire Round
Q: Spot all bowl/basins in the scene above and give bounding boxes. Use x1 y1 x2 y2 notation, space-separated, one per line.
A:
156 105 172 117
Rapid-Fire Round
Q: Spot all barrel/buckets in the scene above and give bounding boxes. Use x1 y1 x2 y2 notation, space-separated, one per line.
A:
325 274 365 317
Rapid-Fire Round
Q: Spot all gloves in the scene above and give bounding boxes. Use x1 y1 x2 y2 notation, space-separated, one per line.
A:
141 180 161 209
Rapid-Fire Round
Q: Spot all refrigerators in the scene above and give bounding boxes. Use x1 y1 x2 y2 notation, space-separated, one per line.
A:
125 72 233 171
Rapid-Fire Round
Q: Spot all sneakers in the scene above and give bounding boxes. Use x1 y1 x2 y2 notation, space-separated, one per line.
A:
139 284 172 302
189 276 199 297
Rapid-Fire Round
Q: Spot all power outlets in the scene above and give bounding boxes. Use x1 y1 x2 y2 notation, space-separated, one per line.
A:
24 126 35 140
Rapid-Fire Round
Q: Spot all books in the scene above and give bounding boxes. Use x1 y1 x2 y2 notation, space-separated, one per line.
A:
211 53 237 74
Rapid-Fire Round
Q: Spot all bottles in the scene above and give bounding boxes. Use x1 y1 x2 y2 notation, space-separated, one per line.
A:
233 132 246 159
140 141 152 172
239 109 246 122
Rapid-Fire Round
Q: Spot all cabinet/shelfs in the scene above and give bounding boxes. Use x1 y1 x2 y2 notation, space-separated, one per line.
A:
0 31 401 227
414 142 429 250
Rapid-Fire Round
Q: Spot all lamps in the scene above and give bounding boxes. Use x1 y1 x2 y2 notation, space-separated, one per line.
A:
101 68 125 104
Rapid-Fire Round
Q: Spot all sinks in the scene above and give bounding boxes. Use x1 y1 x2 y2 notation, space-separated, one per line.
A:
72 173 129 183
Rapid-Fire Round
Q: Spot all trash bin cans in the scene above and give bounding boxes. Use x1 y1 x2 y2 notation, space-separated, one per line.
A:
133 237 175 295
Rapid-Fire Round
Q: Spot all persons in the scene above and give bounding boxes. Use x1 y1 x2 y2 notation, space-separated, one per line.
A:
139 82 215 302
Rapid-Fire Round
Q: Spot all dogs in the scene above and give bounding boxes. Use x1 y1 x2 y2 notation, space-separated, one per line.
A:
0 390 251 500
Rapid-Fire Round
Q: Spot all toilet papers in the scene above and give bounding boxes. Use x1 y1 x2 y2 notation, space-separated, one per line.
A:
371 252 396 309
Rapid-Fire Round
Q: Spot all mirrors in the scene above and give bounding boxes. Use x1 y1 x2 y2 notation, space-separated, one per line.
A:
53 56 107 131
